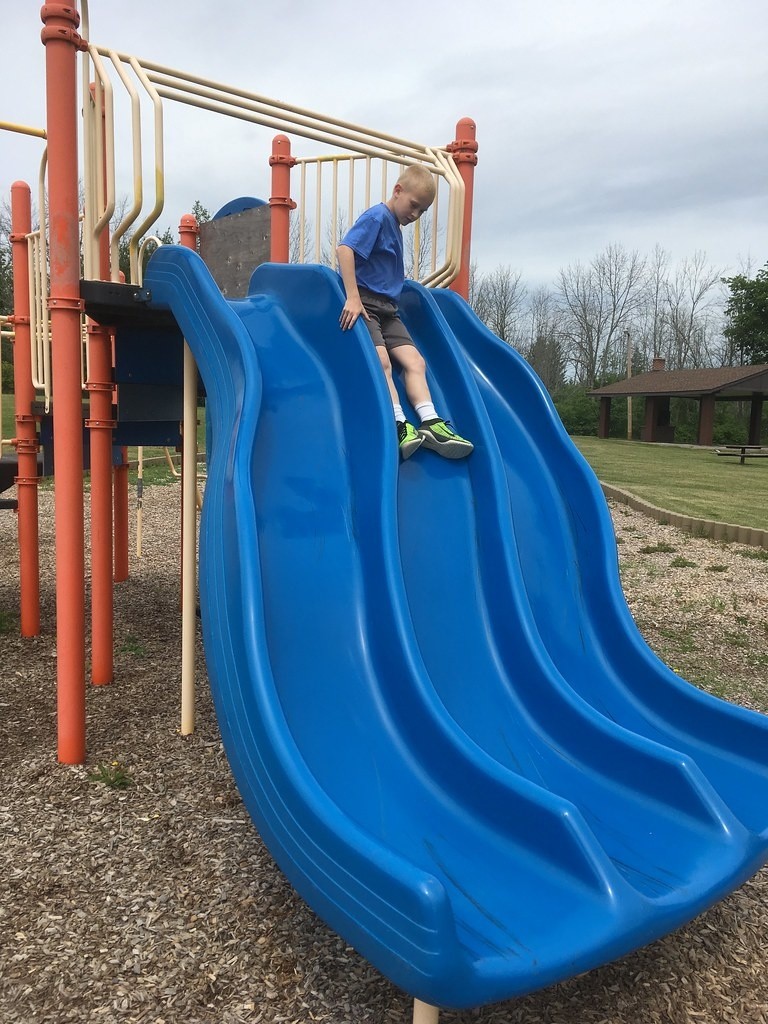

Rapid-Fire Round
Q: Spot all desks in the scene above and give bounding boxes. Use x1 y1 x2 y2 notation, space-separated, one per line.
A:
726 446 762 463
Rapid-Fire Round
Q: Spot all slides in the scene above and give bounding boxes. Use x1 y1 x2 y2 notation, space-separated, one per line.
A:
141 244 768 1012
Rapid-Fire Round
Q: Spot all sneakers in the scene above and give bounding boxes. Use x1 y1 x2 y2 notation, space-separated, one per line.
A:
396 420 426 460
416 417 474 459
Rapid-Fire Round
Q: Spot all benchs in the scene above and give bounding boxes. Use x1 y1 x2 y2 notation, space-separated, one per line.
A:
718 452 768 464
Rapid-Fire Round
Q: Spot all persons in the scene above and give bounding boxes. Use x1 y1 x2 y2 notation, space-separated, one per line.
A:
336 163 475 460
